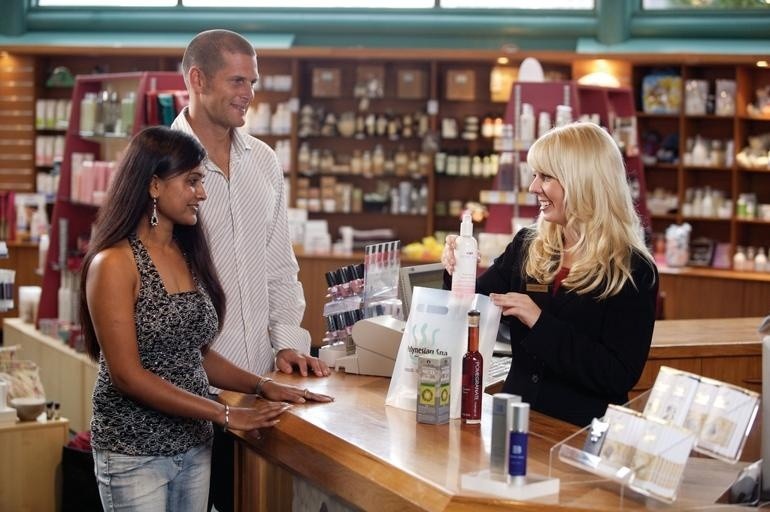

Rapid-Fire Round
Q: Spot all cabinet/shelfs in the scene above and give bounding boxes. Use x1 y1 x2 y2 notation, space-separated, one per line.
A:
0 45 187 435
242 48 769 276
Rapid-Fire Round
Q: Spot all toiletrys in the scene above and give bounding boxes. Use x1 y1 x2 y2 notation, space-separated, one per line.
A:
364 241 402 265
323 260 365 345
507 400 530 489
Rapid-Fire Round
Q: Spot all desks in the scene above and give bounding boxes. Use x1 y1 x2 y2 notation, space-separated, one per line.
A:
0 368 71 511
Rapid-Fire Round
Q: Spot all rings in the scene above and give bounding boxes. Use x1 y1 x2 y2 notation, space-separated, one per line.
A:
304 389 307 399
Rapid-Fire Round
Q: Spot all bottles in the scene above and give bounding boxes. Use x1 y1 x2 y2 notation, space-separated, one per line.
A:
460 310 484 424
507 404 530 478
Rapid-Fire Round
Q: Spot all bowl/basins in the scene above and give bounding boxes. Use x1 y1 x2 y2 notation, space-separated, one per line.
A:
9 397 48 422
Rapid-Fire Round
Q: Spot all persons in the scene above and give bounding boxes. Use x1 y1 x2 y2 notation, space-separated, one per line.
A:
165 28 331 512
440 122 660 431
76 124 334 512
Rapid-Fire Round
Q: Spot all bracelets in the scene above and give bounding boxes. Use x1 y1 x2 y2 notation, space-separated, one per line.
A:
255 377 272 394
222 404 229 433
